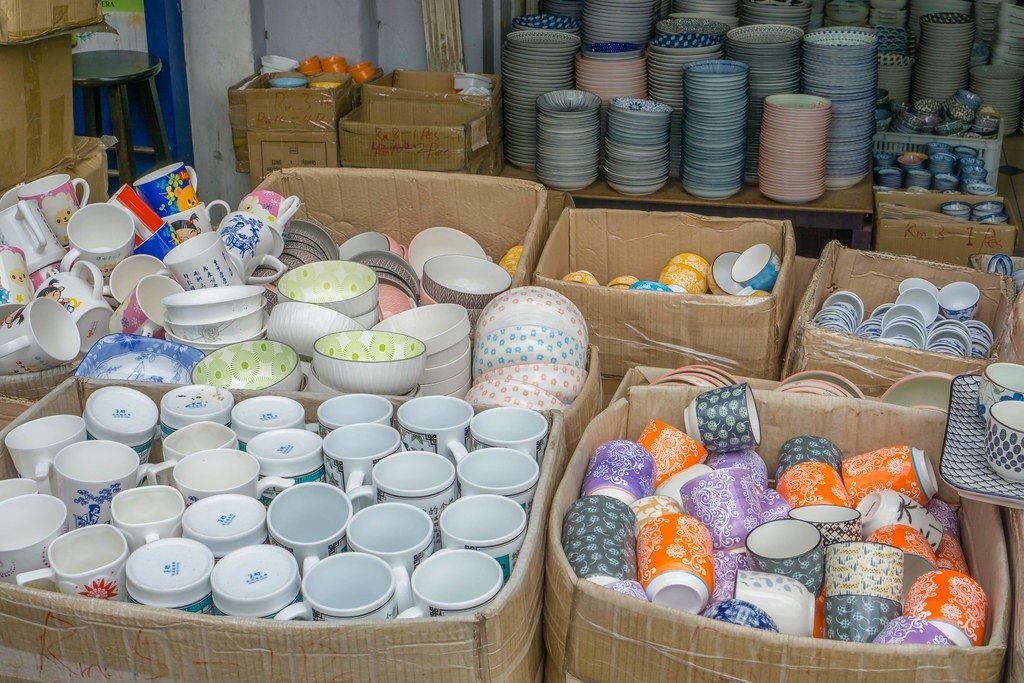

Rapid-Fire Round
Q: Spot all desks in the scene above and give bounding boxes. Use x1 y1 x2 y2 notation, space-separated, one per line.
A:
498 163 875 254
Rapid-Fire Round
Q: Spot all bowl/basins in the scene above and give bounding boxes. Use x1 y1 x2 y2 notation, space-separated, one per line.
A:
161 226 1024 415
939 200 1009 223
268 77 341 88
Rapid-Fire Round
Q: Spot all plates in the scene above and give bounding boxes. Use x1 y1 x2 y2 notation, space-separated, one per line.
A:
500 0 1018 195
938 373 1024 510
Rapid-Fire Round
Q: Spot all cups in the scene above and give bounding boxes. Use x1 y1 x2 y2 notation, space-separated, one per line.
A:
163 231 244 292
0 383 551 626
237 189 302 259
216 211 284 286
985 400 1024 486
977 362 1024 424
0 163 232 377
296 56 376 83
562 382 990 648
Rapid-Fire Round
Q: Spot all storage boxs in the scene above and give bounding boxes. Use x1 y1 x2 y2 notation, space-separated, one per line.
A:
0 375 568 683
779 240 1016 400
361 68 506 177
245 72 353 192
0 0 104 48
0 34 77 191
249 165 551 291
226 64 384 174
875 189 1016 267
530 206 797 381
562 340 605 457
872 118 1004 189
0 135 117 206
338 100 492 174
541 386 1011 683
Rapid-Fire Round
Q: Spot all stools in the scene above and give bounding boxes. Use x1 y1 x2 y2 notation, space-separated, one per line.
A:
72 50 173 190
